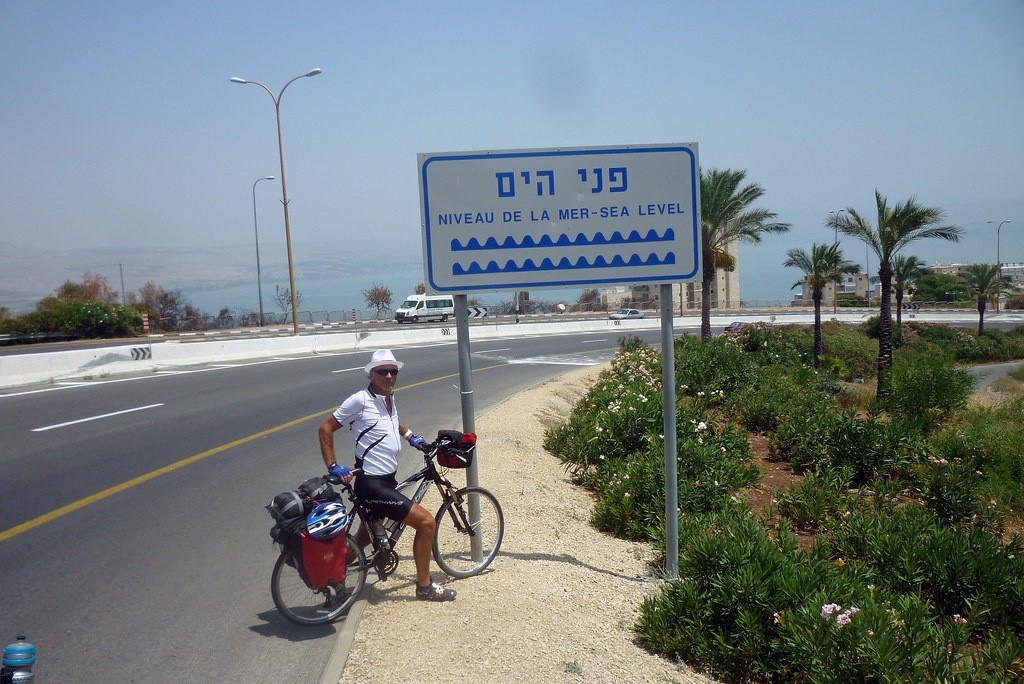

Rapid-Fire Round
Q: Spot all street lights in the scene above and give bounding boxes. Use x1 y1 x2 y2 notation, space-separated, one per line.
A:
829 209 844 315
253 175 275 327
230 66 322 334
987 219 1011 314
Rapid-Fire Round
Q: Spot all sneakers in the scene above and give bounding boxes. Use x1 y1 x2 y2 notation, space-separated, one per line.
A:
415 575 457 601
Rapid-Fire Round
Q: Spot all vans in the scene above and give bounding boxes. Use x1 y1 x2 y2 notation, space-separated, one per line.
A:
394 293 455 322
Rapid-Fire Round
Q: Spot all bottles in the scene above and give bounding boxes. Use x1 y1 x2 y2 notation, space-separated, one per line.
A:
373 518 390 549
3 636 36 683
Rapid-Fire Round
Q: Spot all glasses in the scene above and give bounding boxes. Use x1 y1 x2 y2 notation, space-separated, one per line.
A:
374 368 399 375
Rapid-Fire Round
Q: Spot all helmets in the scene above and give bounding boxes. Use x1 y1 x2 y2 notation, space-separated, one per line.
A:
306 501 350 542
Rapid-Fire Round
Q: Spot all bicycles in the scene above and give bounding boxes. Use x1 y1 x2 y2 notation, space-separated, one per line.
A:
268 429 503 626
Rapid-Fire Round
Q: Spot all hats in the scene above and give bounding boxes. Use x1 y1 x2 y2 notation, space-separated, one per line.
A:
364 349 405 374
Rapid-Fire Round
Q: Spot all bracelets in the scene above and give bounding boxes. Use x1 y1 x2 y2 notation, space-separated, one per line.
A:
404 428 414 439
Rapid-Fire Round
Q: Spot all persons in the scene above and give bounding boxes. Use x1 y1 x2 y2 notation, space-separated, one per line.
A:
319 349 457 602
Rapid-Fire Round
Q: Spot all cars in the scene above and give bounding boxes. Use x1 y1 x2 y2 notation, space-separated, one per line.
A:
609 309 645 319
725 322 749 330
